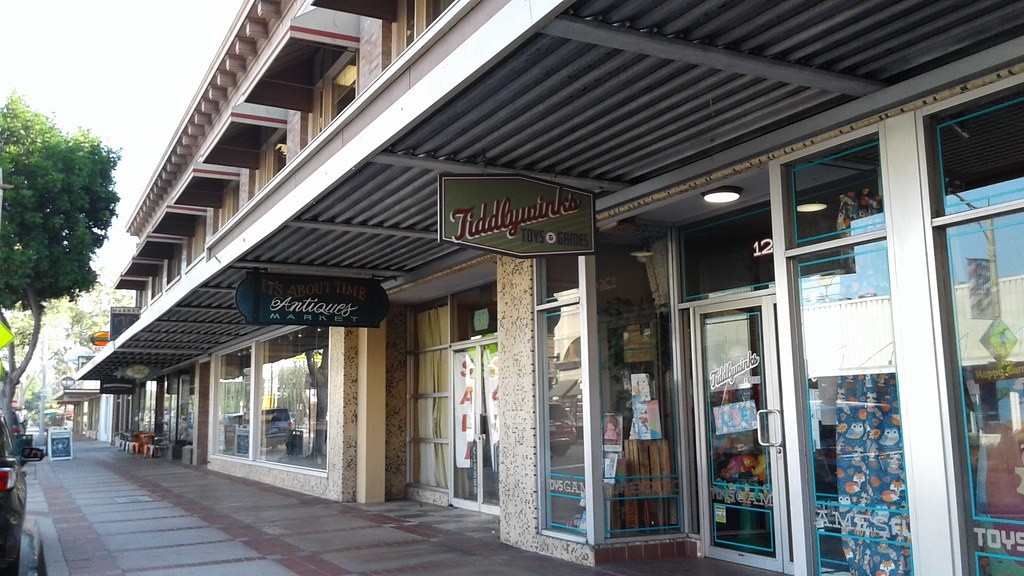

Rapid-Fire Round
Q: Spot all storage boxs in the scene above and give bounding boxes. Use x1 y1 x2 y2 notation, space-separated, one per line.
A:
630 373 663 441
603 412 624 452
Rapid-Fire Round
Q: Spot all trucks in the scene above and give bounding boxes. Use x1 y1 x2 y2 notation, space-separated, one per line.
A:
223 407 293 454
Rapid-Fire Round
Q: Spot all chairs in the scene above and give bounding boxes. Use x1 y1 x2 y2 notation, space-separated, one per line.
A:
113 429 169 459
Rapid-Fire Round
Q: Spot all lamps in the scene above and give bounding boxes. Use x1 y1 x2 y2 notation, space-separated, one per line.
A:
701 186 743 203
630 239 653 263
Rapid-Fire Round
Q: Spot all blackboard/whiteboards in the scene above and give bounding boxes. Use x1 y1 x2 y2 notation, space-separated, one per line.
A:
48 436 73 460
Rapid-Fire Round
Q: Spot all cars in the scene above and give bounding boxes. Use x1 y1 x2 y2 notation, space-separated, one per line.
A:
11 411 28 439
0 409 46 576
549 402 578 458
